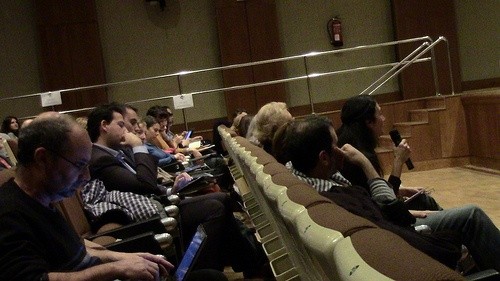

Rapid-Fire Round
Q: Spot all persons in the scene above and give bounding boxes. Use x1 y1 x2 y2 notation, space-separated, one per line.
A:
0 102 274 281
230 94 500 276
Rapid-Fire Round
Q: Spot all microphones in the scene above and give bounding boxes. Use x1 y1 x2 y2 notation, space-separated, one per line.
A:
389 130 414 170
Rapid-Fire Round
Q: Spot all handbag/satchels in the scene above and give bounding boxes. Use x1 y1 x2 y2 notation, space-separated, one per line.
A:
160 161 185 173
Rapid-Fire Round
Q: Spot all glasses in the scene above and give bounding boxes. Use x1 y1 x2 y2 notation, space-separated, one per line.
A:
33 145 90 171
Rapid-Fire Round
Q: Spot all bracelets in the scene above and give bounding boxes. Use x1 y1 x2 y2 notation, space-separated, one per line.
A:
183 148 189 154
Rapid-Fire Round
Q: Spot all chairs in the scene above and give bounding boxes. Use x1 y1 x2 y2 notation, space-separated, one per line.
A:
0 124 471 281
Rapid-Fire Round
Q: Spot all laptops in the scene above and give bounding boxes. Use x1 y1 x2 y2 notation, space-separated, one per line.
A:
174 224 207 281
184 130 193 139
